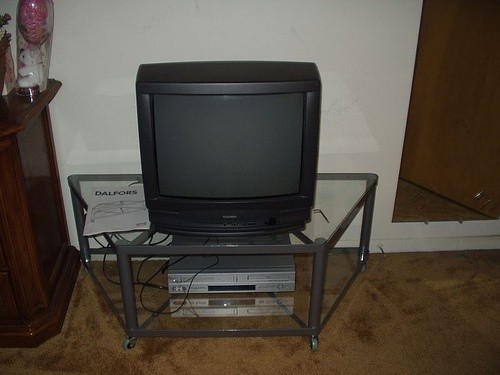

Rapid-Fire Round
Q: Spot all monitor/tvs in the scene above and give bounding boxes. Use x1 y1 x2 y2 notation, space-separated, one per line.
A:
136 61 322 236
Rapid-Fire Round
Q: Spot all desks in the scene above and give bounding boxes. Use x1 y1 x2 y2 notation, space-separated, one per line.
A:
67 172 379 352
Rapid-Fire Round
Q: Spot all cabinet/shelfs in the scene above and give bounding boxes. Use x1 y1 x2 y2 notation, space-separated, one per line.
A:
0 78 81 349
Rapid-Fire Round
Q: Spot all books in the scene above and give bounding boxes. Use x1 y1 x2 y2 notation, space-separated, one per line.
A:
82 184 151 237
